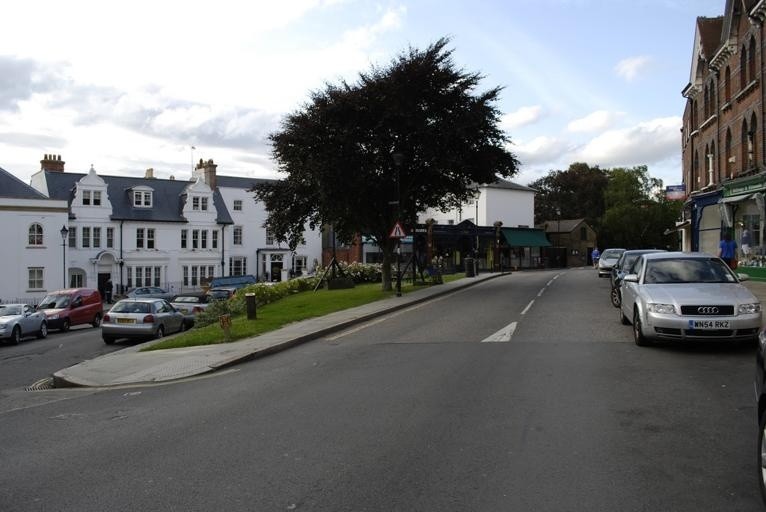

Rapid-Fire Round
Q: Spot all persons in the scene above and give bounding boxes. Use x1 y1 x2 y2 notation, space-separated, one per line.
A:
590 246 601 269
719 230 740 270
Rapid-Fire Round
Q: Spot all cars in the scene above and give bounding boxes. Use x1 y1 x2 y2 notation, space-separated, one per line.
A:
598 247 628 278
100 284 242 345
0 304 49 345
753 326 766 508
619 250 765 351
611 249 670 310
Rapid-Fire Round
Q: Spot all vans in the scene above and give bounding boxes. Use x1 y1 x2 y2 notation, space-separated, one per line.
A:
209 275 257 290
34 288 104 332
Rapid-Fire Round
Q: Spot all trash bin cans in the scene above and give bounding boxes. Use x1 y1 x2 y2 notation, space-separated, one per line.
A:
464 258 474 277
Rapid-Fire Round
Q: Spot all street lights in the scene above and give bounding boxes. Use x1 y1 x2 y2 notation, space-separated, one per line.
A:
555 205 562 269
58 223 70 290
390 146 403 299
472 184 483 276
